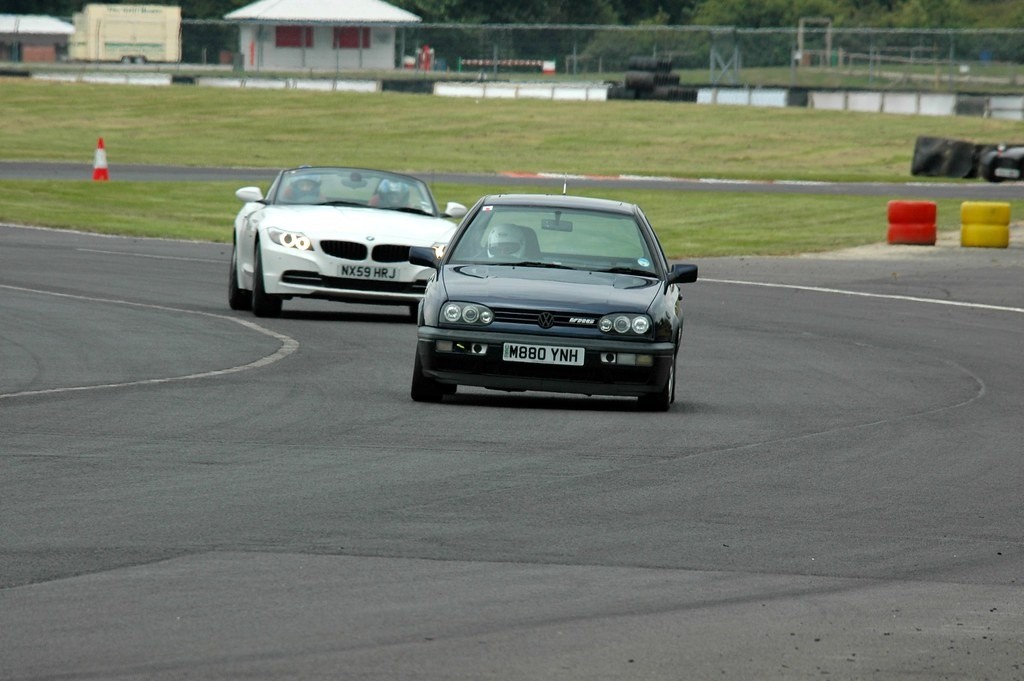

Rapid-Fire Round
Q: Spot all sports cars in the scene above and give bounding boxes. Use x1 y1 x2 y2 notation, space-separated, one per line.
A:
227 166 471 323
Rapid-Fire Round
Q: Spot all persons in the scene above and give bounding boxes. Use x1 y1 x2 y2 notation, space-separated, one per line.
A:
283 162 324 205
372 175 410 209
486 222 526 263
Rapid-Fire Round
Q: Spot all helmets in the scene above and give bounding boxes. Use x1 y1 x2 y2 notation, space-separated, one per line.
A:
487 223 526 257
378 178 409 208
290 165 321 198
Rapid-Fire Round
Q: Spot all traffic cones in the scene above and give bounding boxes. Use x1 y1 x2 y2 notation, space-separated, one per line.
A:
92 137 109 181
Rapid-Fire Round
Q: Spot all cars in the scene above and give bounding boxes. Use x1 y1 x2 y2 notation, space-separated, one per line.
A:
411 194 698 412
